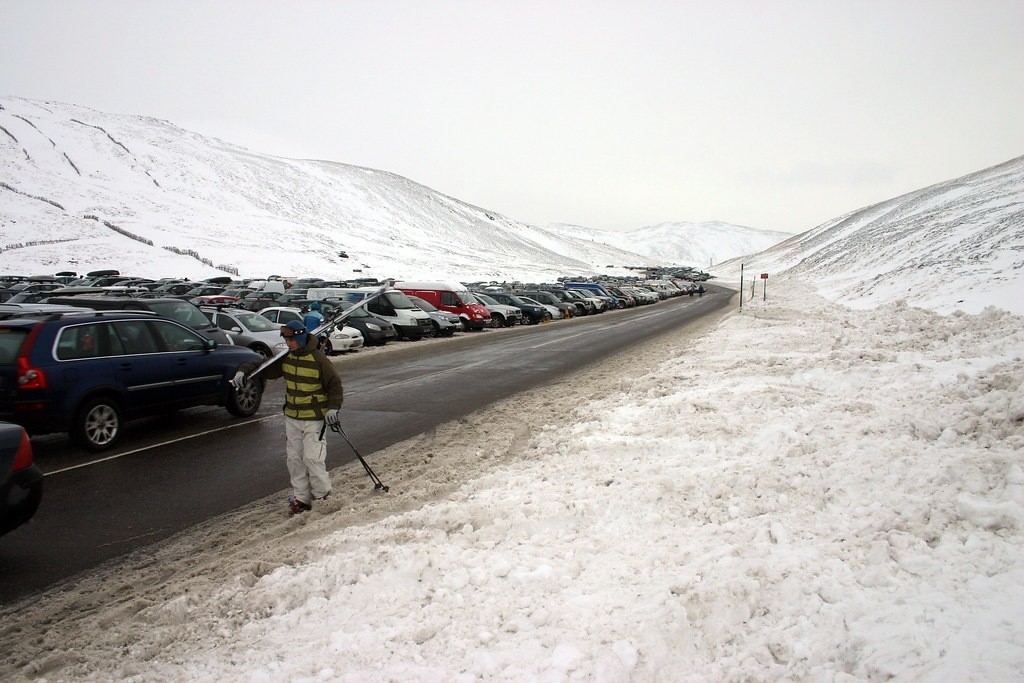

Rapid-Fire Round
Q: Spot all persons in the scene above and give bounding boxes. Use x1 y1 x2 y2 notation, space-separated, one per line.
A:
303 301 325 350
689 281 704 296
232 320 343 517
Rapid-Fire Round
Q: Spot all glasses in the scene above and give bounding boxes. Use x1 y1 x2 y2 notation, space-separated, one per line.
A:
280 326 305 337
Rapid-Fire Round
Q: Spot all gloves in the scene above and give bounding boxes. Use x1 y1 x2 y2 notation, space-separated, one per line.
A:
231 371 247 389
325 409 339 425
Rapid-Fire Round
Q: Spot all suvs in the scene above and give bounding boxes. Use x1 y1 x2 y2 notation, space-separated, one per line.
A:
0 309 266 452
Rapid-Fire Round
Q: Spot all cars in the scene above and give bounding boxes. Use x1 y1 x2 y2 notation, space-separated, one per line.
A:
0 421 43 537
0 270 707 360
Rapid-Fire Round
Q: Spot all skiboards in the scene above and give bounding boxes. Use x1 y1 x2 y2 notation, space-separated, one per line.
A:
230 279 396 383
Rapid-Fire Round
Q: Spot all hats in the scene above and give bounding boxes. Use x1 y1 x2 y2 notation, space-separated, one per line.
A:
311 302 320 311
283 321 306 347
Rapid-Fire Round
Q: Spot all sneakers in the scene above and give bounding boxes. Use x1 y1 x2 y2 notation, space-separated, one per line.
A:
284 500 311 517
